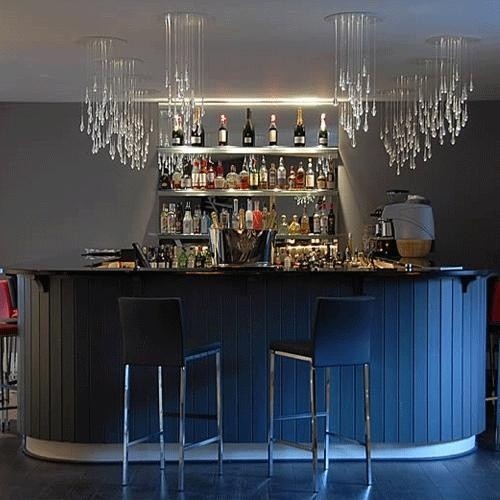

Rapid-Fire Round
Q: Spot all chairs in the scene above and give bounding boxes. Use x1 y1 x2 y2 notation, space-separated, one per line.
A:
118 297 223 492
485 279 500 452
267 296 376 493
0 273 18 433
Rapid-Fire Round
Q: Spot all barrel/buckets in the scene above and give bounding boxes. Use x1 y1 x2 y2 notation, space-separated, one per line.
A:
206 226 278 270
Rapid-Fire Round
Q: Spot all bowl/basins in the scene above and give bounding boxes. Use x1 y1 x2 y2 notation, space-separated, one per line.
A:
384 190 431 205
398 258 432 268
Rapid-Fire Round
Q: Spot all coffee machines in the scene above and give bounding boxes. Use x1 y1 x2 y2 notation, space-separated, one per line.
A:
371 203 434 257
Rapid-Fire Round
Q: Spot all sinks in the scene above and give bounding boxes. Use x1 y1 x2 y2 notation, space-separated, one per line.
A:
94 260 135 269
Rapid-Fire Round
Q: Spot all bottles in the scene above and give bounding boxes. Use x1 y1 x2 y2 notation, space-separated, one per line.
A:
243 109 255 147
269 115 277 144
147 244 215 268
170 116 183 146
159 156 335 191
190 107 204 147
217 115 229 147
273 265 376 272
317 113 328 146
294 107 306 147
277 232 368 267
160 198 334 235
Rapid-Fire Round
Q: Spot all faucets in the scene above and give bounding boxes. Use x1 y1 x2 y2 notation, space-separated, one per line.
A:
132 242 151 268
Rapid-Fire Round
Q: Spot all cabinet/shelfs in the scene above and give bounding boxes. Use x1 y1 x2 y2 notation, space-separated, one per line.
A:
157 147 340 240
